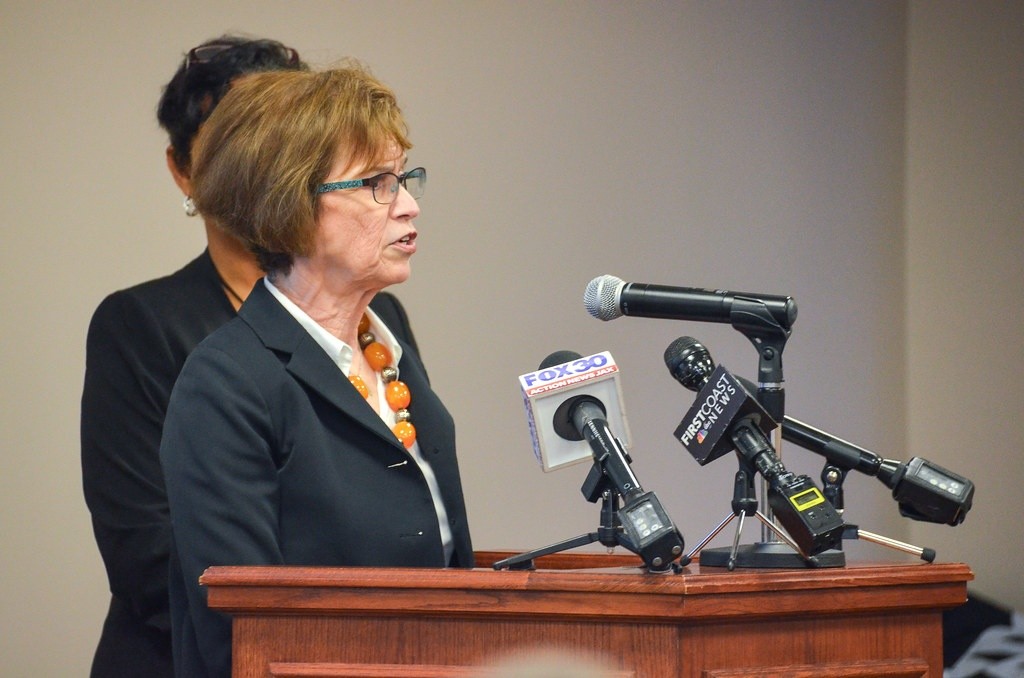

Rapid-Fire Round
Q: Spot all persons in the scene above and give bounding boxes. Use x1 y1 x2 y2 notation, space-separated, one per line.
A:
79 37 308 678
162 66 475 678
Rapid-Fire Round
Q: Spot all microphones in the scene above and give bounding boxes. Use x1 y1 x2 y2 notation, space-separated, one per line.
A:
583 275 798 331
780 413 882 476
663 336 795 490
517 351 645 506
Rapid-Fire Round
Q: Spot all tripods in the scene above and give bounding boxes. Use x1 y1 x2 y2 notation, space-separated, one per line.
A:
677 423 845 571
493 438 685 575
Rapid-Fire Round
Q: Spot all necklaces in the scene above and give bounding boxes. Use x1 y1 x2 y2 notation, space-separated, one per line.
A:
343 309 417 452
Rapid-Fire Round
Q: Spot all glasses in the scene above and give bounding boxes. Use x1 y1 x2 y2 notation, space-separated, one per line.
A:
315 167 426 205
186 44 300 72
